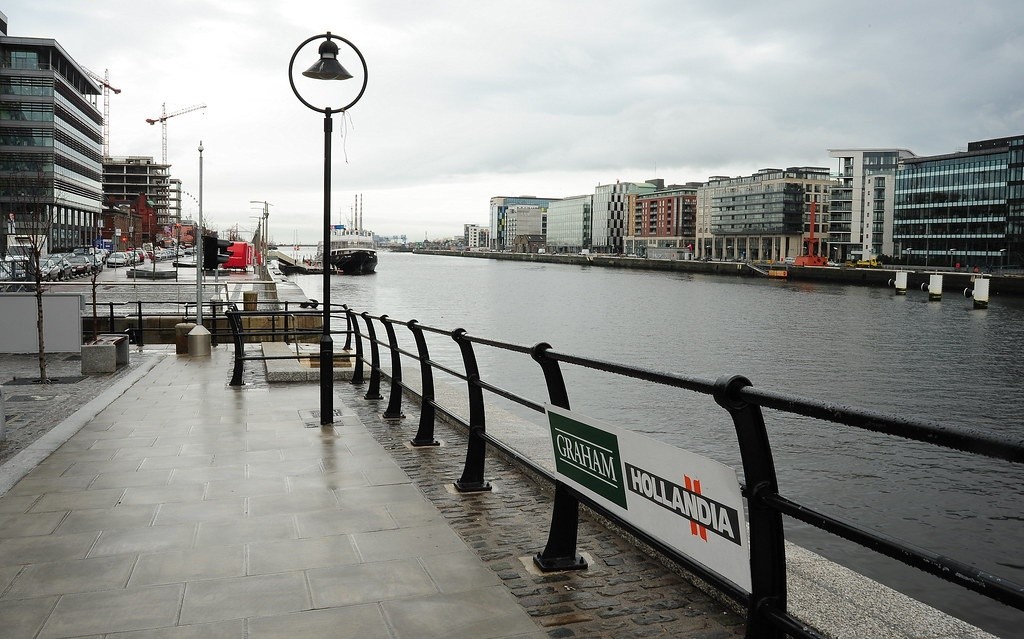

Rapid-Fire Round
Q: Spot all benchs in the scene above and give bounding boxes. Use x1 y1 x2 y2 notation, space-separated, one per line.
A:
80 334 129 373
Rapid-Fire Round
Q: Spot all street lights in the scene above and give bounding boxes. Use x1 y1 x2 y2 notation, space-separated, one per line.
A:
286 31 369 430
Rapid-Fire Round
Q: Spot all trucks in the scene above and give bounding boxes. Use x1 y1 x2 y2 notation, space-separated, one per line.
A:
856 258 883 268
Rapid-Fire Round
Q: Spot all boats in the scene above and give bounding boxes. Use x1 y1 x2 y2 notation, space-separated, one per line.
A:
316 194 378 274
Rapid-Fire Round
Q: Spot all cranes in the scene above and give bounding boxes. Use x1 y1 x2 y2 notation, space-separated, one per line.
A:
79 63 121 157
144 101 208 223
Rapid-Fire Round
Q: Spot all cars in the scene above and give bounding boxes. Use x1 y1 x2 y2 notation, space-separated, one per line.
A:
108 249 145 267
0 253 105 281
149 247 193 261
826 261 840 267
784 257 796 265
